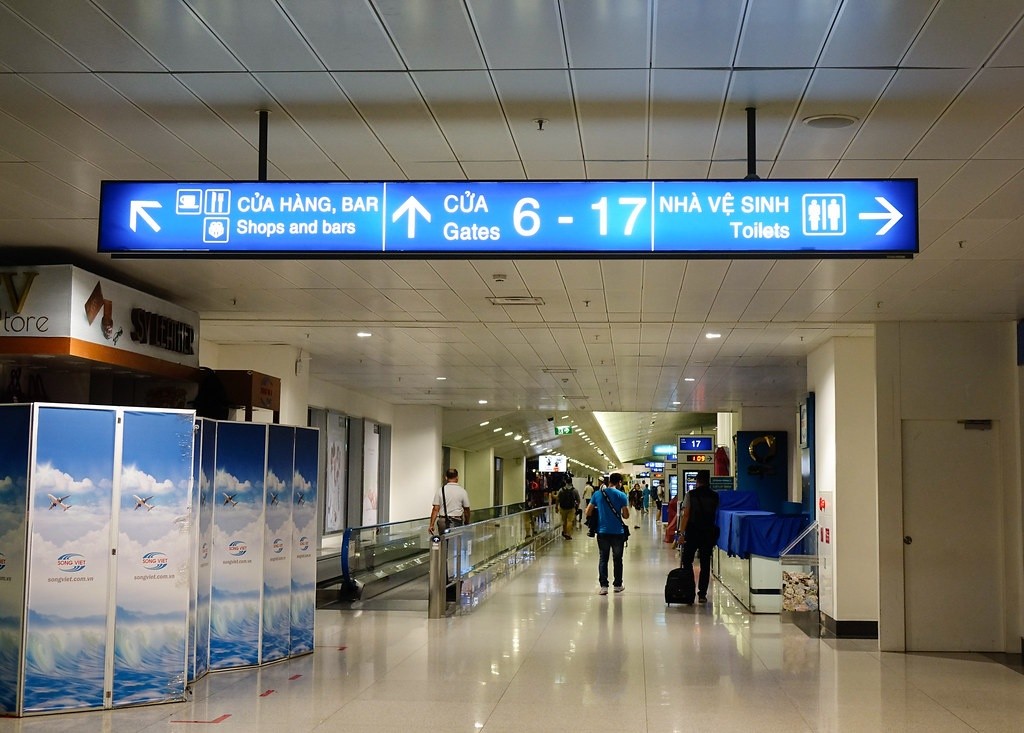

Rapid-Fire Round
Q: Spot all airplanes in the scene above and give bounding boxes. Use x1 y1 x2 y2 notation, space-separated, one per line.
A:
222 492 239 508
297 492 306 505
132 494 155 513
269 492 280 506
46 493 72 512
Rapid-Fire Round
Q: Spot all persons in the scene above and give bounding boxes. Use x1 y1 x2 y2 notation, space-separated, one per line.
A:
585 473 629 594
527 476 608 540
656 479 665 521
678 472 720 603
428 469 470 536
620 480 651 529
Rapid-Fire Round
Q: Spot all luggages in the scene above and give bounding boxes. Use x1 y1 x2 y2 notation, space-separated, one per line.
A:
665 544 696 607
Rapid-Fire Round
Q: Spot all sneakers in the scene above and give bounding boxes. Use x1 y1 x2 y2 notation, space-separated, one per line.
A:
599 586 608 595
614 586 625 591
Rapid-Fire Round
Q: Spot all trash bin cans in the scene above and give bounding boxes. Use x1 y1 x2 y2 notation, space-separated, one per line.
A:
779 520 821 622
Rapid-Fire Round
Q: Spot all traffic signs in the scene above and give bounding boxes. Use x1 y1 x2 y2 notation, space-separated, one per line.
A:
96 176 919 260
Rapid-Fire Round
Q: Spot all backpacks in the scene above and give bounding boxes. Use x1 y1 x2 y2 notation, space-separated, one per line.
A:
559 487 576 510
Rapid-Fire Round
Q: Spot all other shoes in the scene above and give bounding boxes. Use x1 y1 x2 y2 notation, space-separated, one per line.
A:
699 596 707 602
566 536 572 540
562 531 568 538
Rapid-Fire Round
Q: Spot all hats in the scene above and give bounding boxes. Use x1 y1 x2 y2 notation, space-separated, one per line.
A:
694 470 710 479
598 476 604 482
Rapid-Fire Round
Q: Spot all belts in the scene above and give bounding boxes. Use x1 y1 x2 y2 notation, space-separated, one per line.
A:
439 515 461 518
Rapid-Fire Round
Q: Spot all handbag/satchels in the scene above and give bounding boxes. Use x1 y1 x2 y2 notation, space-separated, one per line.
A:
445 518 462 533
623 524 631 543
713 525 720 545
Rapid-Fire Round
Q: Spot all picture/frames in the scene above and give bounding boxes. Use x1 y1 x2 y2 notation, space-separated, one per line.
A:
799 398 809 449
